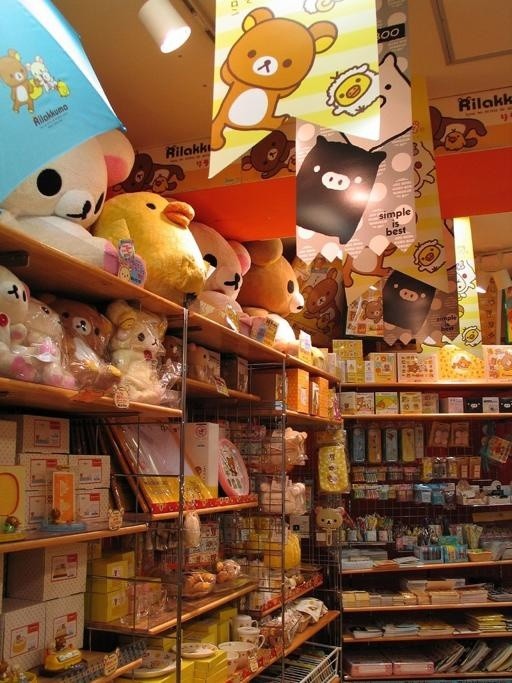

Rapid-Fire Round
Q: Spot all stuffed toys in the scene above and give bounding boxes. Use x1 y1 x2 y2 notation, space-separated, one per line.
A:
0 127 307 404
3 128 306 404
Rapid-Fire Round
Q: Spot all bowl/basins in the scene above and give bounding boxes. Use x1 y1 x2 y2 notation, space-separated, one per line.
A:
217 639 257 677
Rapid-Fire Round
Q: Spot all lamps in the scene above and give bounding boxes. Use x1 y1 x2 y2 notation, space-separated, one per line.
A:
138 0 192 53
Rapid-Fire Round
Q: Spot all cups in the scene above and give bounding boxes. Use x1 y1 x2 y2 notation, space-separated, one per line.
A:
237 626 265 652
231 614 259 642
116 584 168 624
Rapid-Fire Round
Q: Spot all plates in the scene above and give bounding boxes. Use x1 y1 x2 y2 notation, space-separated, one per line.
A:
172 641 219 659
121 648 182 678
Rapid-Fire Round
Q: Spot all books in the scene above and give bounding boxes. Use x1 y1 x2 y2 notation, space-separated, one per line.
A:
342 574 511 677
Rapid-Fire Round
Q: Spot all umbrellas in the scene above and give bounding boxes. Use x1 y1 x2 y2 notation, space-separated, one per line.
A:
0 1 128 205
